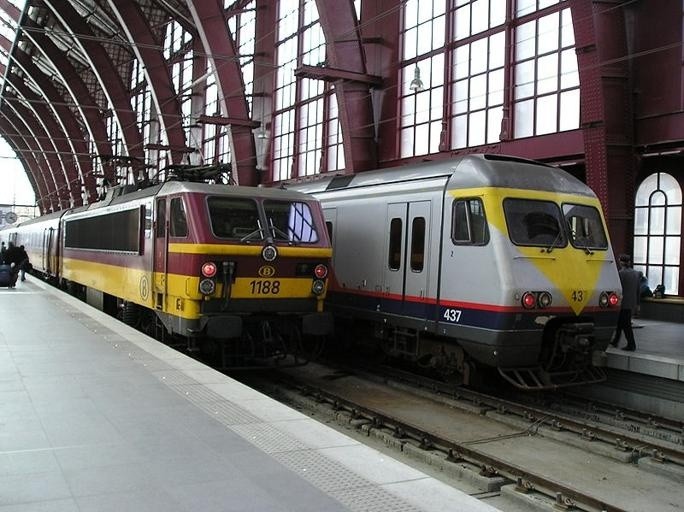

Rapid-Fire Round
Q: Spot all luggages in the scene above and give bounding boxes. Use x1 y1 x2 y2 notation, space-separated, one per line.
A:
0 263 13 289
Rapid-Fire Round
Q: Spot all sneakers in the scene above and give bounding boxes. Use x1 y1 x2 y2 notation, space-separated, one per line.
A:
609 342 619 349
620 344 637 353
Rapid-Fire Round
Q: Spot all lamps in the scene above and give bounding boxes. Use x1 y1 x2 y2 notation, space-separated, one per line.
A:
409 1 425 93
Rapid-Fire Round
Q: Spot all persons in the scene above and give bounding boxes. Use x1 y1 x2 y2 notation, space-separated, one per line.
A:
5 242 21 288
20 245 28 281
1 241 7 260
609 253 641 352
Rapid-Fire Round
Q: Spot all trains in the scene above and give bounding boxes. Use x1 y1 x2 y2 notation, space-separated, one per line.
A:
276 153 624 390
2 181 334 370
